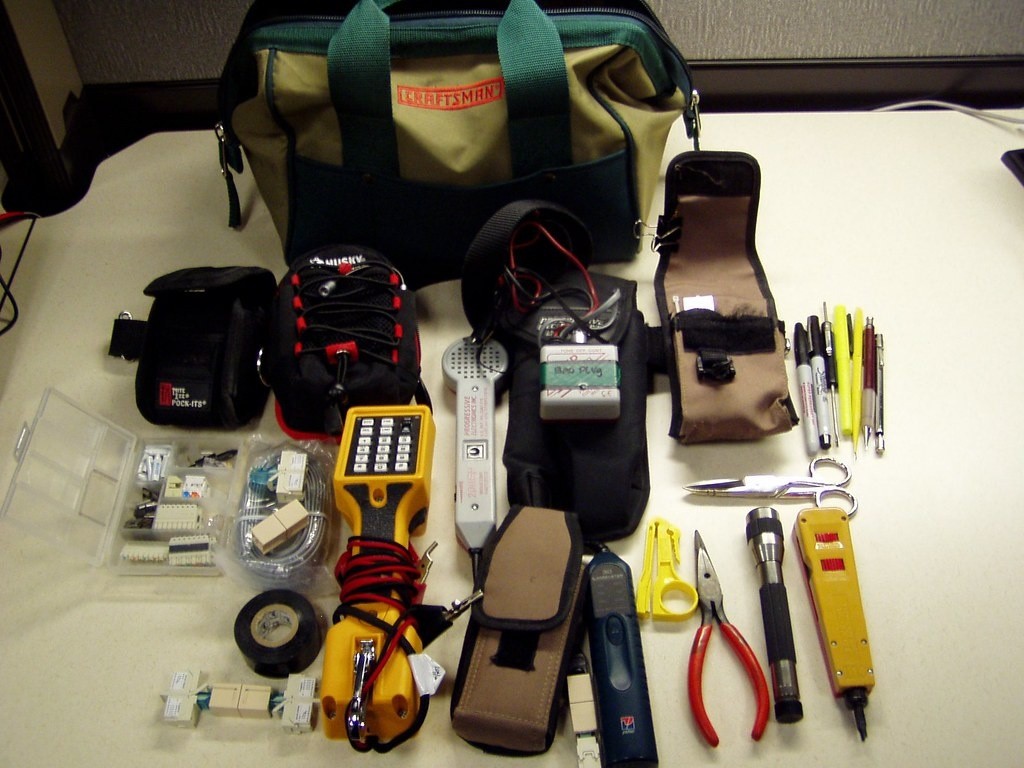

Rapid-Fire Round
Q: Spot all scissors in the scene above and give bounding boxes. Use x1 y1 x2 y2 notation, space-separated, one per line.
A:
681 456 858 519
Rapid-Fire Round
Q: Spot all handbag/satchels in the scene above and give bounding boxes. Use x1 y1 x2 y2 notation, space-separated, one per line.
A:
214 0 702 291
449 506 588 757
109 266 279 431
653 150 799 444
461 200 668 545
277 245 433 444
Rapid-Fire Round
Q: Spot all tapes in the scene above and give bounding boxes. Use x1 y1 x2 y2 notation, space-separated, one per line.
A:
233 588 323 679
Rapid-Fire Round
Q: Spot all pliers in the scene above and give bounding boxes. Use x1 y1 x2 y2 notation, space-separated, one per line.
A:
688 530 771 748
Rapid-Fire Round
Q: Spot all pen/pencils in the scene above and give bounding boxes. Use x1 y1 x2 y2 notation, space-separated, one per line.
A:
793 302 886 457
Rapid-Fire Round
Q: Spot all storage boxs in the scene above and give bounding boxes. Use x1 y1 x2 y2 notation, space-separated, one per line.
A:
1 386 244 579
